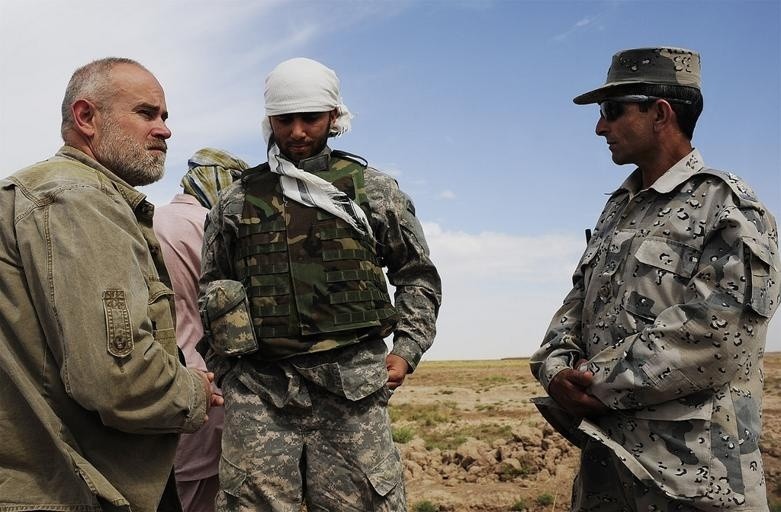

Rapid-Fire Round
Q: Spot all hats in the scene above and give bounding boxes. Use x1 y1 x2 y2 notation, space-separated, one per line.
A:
573 48 700 106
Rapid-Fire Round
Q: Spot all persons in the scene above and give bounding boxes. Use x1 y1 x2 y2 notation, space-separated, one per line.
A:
528 47 781 512
150 150 250 512
197 58 442 511
0 57 224 512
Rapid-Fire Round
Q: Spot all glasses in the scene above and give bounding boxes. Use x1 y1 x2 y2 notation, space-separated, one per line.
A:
598 94 690 122
